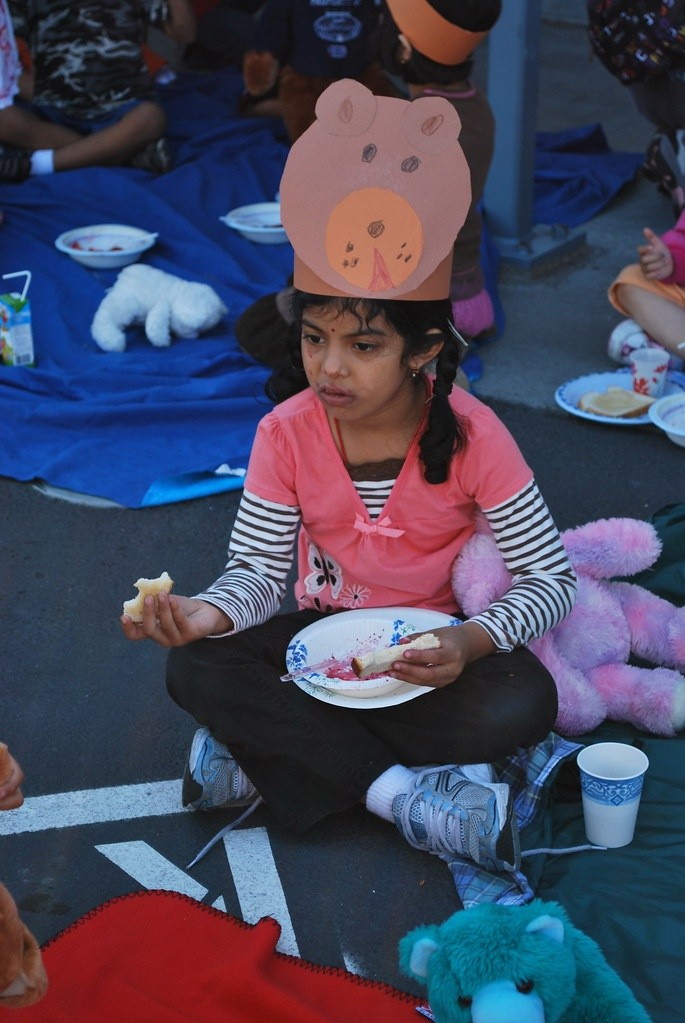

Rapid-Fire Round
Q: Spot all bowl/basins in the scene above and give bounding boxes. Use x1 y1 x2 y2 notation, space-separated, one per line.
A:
648 391 685 447
294 617 419 698
224 202 291 244
54 224 157 269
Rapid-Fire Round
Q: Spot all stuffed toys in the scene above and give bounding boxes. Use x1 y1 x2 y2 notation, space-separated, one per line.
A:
394 897 652 1023
90 261 228 355
450 514 685 736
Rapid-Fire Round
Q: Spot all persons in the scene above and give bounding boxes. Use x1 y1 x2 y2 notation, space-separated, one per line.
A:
607 203 685 358
118 78 576 871
0 0 506 395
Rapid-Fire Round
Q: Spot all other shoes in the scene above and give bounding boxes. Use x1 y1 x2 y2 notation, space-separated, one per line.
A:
0 151 31 183
607 319 685 370
128 141 171 173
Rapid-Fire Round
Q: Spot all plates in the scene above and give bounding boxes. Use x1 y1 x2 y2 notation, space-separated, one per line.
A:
554 371 685 425
284 605 465 710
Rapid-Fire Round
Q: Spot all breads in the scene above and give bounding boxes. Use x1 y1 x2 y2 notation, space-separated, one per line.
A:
123 571 174 623
578 386 656 419
350 633 442 679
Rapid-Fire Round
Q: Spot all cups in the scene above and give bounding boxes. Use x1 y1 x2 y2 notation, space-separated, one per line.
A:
576 741 651 847
631 349 669 399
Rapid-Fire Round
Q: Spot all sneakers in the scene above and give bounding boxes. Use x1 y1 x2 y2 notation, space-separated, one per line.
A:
392 763 608 873
181 727 268 870
